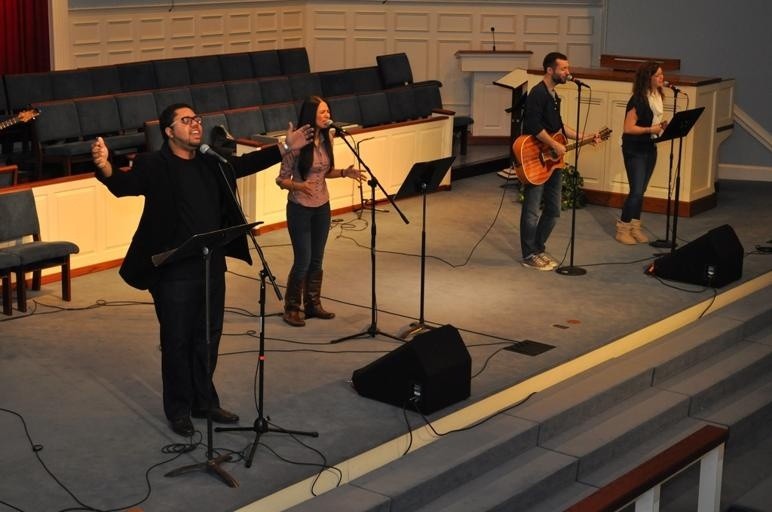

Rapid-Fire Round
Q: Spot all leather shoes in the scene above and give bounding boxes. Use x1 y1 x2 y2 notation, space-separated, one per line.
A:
191 406 238 423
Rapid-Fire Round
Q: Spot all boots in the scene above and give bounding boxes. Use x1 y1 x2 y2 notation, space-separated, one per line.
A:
303 271 334 318
632 218 648 242
616 218 636 244
283 290 305 326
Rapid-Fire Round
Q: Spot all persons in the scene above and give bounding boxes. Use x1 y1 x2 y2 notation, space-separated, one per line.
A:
275 96 366 328
519 52 602 270
615 61 669 244
90 104 315 437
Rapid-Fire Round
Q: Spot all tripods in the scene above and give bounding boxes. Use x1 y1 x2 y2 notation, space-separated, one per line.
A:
398 153 458 340
649 106 707 253
163 214 265 489
330 134 406 345
214 163 319 469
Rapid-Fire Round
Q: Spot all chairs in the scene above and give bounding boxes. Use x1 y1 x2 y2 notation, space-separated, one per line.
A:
0 187 80 316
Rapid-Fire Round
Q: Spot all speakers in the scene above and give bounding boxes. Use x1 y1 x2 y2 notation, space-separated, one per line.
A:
654 224 744 288
351 324 472 416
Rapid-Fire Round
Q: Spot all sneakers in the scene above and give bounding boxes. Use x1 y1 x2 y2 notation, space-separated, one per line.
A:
538 253 558 267
522 256 553 271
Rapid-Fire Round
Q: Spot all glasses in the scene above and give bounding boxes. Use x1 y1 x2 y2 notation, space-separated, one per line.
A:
170 115 203 125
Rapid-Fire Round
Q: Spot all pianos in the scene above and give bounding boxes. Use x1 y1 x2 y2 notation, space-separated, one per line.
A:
251 120 362 143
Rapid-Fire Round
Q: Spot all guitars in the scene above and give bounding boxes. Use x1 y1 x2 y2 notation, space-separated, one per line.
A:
514 125 612 185
0 106 40 130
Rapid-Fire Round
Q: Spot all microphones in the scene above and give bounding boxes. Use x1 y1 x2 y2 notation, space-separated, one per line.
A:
200 143 228 164
326 120 347 133
663 81 688 95
491 27 495 51
566 74 591 88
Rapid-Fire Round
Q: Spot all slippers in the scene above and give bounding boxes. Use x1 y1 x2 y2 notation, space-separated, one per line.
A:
173 415 194 437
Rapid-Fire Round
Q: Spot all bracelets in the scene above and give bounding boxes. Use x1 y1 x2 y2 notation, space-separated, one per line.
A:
340 169 345 178
282 142 291 152
